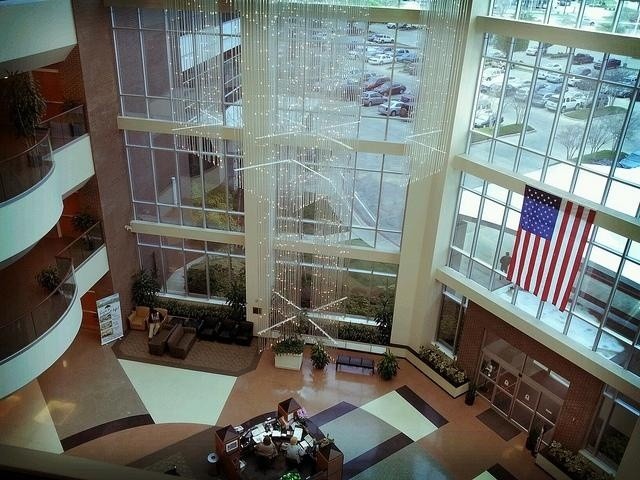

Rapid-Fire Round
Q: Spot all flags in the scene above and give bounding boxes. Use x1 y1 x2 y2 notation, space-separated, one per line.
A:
506 184 597 314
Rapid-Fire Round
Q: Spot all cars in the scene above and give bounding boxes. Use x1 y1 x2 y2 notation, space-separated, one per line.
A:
561 14 595 26
476 101 491 112
480 45 640 113
377 101 409 116
314 17 421 106
475 114 504 128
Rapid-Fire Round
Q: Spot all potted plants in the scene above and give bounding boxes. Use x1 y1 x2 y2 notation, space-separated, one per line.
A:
72 213 99 251
376 347 401 380
60 96 82 137
0 69 47 167
271 337 332 370
31 264 62 296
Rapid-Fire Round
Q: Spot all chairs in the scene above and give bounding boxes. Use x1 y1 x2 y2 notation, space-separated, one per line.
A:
197 318 253 347
128 306 168 333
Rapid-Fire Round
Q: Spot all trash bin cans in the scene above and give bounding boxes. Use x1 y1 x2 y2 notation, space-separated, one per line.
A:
207 452 220 476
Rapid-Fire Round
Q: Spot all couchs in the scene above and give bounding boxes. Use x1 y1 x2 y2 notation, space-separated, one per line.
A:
148 324 197 360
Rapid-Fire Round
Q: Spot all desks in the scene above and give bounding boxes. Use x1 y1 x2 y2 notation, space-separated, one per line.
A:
215 398 343 479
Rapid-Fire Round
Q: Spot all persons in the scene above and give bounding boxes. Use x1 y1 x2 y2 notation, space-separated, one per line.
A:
254 434 279 459
284 436 307 462
499 251 512 282
146 309 163 342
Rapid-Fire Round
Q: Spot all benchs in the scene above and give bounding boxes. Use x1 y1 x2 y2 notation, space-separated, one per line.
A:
336 355 374 376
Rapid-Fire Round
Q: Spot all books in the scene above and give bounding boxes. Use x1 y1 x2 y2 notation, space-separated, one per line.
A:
251 421 317 449
